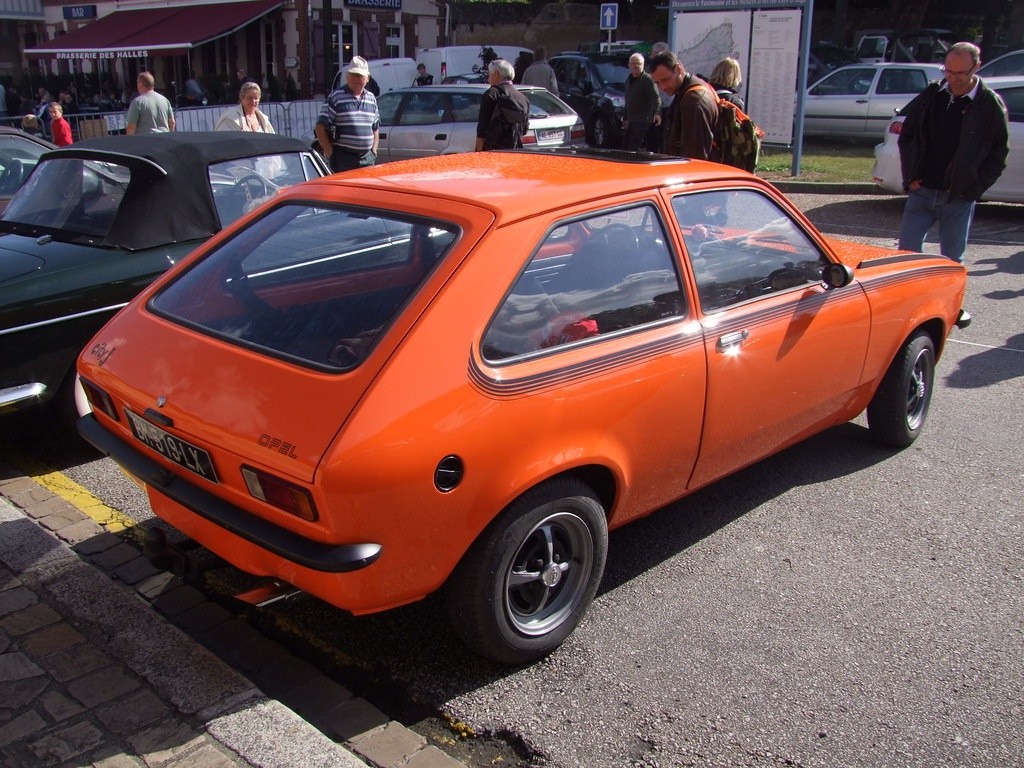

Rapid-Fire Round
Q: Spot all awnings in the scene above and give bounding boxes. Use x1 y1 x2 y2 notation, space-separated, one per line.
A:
24 0 297 107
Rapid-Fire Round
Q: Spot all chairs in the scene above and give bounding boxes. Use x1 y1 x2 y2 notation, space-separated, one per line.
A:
490 276 560 341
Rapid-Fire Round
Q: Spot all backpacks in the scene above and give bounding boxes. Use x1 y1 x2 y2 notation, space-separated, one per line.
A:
684 74 764 176
486 85 525 149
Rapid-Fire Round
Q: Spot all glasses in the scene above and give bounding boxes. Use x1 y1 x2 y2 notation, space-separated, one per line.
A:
940 63 976 79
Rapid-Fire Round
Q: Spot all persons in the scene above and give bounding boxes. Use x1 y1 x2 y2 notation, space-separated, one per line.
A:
475 60 531 153
414 64 436 102
520 45 560 115
0 70 255 148
622 42 745 227
898 42 1010 325
315 55 382 174
215 82 289 182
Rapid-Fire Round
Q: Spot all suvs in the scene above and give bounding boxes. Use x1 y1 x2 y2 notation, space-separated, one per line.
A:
548 51 632 146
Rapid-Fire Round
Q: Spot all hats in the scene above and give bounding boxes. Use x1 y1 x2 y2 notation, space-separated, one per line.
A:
347 55 368 77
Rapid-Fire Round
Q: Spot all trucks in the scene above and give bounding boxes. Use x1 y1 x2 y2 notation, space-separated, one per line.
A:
331 57 422 106
415 46 537 84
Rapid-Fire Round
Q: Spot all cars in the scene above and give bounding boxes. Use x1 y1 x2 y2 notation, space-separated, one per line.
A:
302 83 590 165
0 124 235 217
582 29 983 88
77 147 972 667
975 49 1024 77
872 75 1024 205
440 73 487 84
794 62 946 142
0 131 455 452
